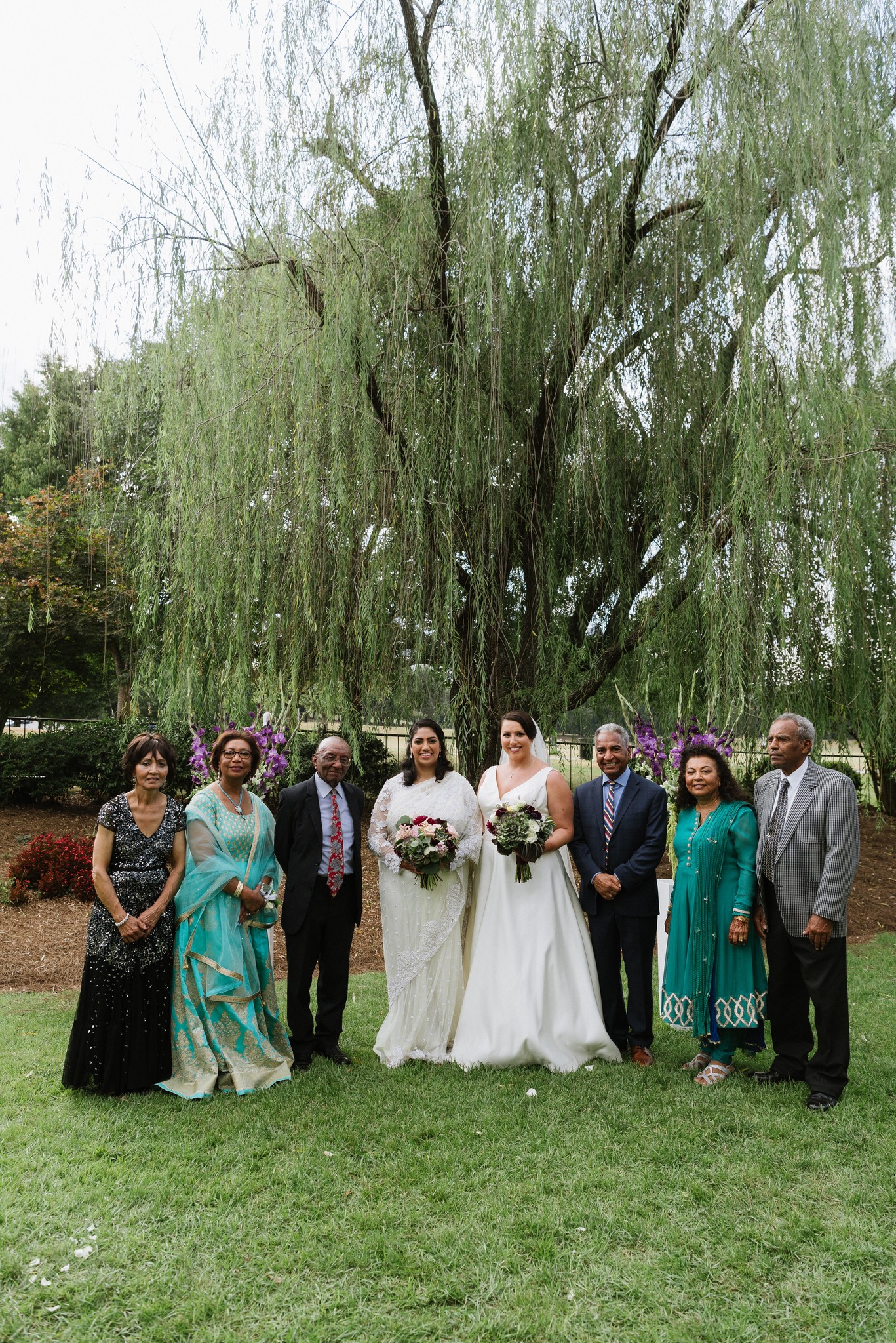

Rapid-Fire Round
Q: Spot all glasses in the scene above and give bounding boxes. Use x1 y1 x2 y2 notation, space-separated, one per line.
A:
316 753 352 767
221 748 252 760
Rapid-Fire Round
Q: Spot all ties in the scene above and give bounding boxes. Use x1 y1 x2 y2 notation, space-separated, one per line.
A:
326 789 345 897
602 781 616 874
762 779 791 884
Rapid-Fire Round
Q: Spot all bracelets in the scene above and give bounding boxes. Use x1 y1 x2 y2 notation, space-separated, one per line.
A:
115 912 130 927
733 915 750 924
261 882 265 886
233 881 244 898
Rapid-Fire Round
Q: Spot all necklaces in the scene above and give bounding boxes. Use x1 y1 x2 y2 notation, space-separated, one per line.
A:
696 796 720 827
507 753 532 777
217 778 247 820
416 775 421 782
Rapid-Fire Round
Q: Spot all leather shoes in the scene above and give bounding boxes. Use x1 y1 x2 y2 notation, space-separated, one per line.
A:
630 1044 653 1067
292 1047 312 1069
806 1090 837 1109
746 1068 787 1085
315 1044 351 1065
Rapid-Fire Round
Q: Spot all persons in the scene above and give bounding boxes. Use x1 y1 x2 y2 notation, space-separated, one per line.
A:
476 711 578 1071
747 712 861 1112
62 732 187 1096
659 744 767 1088
274 736 365 1069
567 723 667 1066
367 719 483 1070
151 729 295 1101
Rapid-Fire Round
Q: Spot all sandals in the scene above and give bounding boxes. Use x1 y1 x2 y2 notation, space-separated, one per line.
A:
690 1060 735 1087
681 1053 711 1073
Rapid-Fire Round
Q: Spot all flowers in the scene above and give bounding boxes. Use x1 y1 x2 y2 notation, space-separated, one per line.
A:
486 799 556 883
392 814 468 890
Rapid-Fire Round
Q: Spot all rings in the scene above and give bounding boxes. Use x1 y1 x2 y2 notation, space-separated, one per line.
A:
246 912 251 916
738 937 744 941
255 909 259 913
